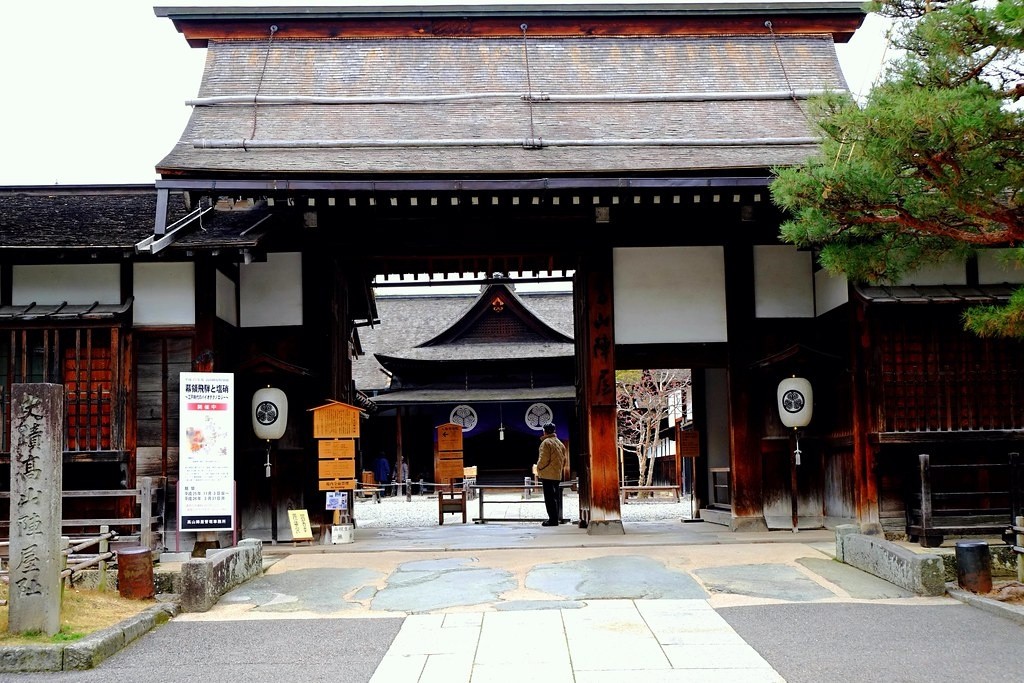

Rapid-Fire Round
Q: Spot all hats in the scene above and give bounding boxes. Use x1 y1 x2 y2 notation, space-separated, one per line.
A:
543 423 555 432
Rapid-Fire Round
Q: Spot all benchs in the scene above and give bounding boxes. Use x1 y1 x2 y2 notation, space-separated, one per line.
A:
620 486 680 505
353 489 386 504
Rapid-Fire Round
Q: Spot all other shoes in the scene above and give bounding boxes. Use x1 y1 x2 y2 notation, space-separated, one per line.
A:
542 520 558 526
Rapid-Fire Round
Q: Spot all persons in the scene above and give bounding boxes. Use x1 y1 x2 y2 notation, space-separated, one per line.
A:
392 455 409 496
370 450 391 497
537 423 567 526
